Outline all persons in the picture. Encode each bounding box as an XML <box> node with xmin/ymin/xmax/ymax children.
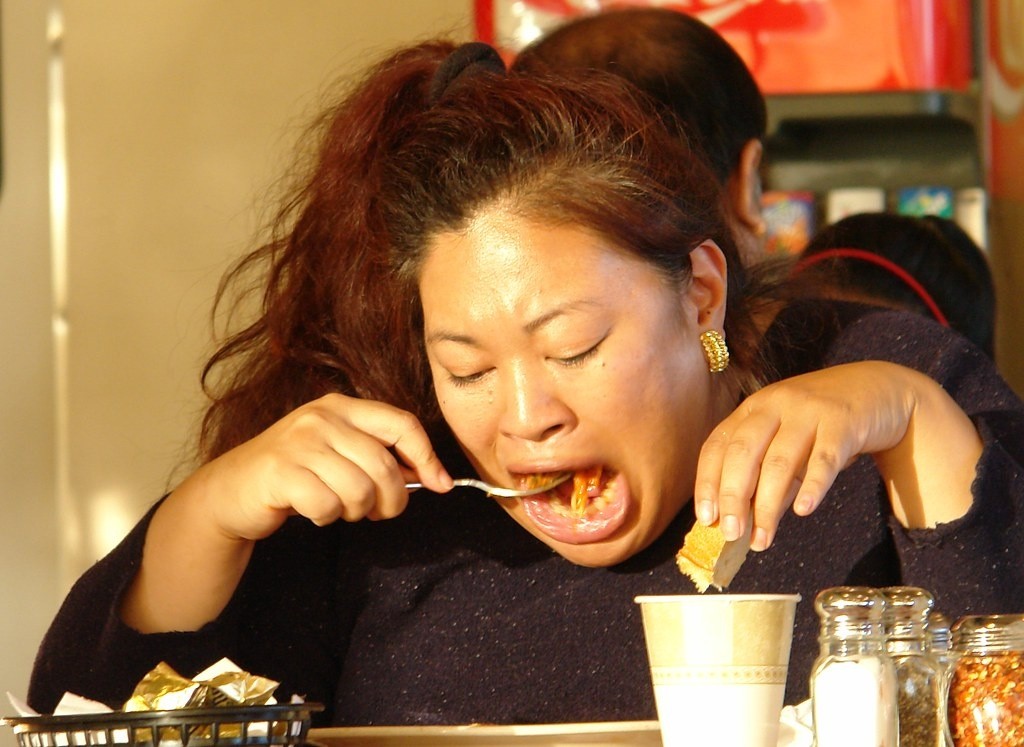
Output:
<box><xmin>26</xmin><ymin>8</ymin><xmax>1023</xmax><ymax>726</ymax></box>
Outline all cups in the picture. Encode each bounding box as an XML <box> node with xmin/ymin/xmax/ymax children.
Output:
<box><xmin>634</xmin><ymin>592</ymin><xmax>803</xmax><ymax>747</ymax></box>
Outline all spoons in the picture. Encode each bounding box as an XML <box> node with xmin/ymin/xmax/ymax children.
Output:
<box><xmin>401</xmin><ymin>472</ymin><xmax>576</xmax><ymax>500</ymax></box>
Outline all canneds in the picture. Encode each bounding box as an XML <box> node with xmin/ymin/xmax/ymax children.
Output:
<box><xmin>760</xmin><ymin>191</ymin><xmax>813</xmax><ymax>255</ymax></box>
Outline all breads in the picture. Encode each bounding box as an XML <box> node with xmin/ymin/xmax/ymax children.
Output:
<box><xmin>676</xmin><ymin>519</ymin><xmax>755</xmax><ymax>593</ymax></box>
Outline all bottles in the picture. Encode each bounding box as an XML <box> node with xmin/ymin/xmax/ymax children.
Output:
<box><xmin>880</xmin><ymin>586</ymin><xmax>939</xmax><ymax>747</ymax></box>
<box><xmin>811</xmin><ymin>588</ymin><xmax>899</xmax><ymax>747</ymax></box>
<box><xmin>945</xmin><ymin>615</ymin><xmax>1024</xmax><ymax>746</ymax></box>
